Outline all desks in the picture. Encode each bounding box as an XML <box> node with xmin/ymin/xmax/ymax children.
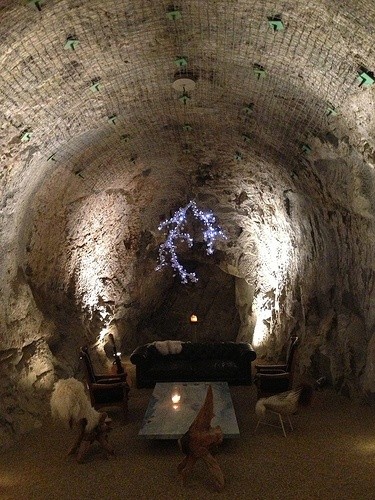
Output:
<box><xmin>137</xmin><ymin>382</ymin><xmax>240</xmax><ymax>440</ymax></box>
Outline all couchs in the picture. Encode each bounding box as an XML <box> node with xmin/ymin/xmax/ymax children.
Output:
<box><xmin>129</xmin><ymin>340</ymin><xmax>257</xmax><ymax>390</ymax></box>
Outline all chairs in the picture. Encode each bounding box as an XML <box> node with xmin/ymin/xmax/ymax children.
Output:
<box><xmin>77</xmin><ymin>346</ymin><xmax>131</xmax><ymax>425</ymax></box>
<box><xmin>254</xmin><ymin>387</ymin><xmax>303</xmax><ymax>439</ymax></box>
<box><xmin>254</xmin><ymin>336</ymin><xmax>302</xmax><ymax>401</ymax></box>
